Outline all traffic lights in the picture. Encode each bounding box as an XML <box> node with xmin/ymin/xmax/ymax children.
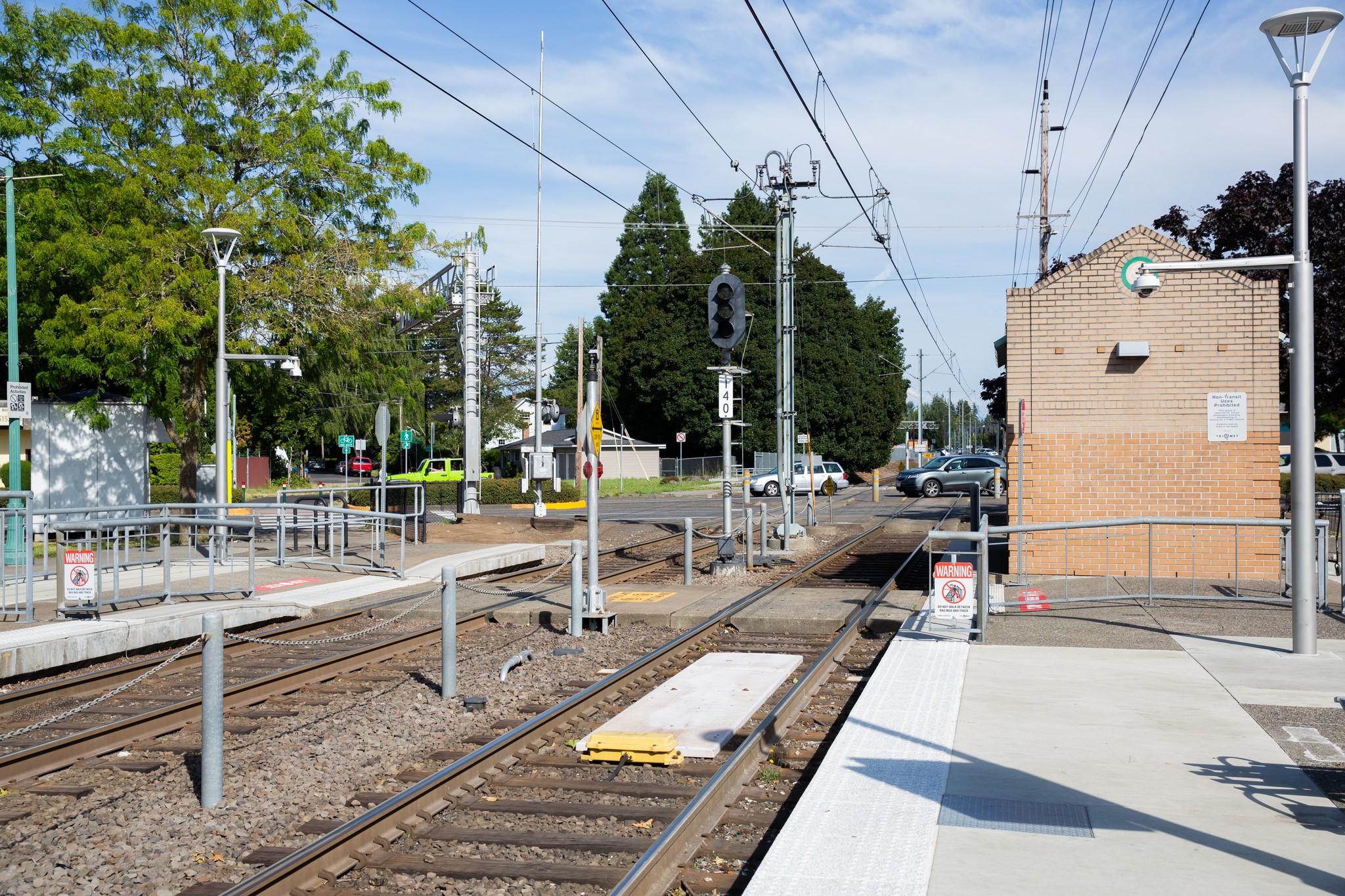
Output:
<box><xmin>708</xmin><ymin>273</ymin><xmax>745</xmax><ymax>349</ymax></box>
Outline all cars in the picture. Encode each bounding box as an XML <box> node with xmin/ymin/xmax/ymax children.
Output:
<box><xmin>305</xmin><ymin>457</ymin><xmax>325</xmax><ymax>470</ymax></box>
<box><xmin>335</xmin><ymin>456</ymin><xmax>380</xmax><ymax>477</ymax></box>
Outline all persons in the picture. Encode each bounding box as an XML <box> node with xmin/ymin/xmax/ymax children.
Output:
<box><xmin>428</xmin><ymin>461</ymin><xmax>445</xmax><ymax>471</ymax></box>
<box><xmin>794</xmin><ymin>465</ymin><xmax>804</xmax><ymax>474</ymax></box>
<box><xmin>954</xmin><ymin>462</ymin><xmax>962</xmax><ymax>469</ymax></box>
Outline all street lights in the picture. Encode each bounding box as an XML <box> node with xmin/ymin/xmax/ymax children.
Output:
<box><xmin>1259</xmin><ymin>5</ymin><xmax>1345</xmax><ymax>657</ymax></box>
<box><xmin>200</xmin><ymin>226</ymin><xmax>242</xmax><ymax>563</ymax></box>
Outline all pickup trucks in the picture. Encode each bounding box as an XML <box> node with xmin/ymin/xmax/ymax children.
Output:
<box><xmin>386</xmin><ymin>457</ymin><xmax>501</xmax><ymax>483</ymax></box>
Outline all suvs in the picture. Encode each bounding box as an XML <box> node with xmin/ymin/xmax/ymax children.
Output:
<box><xmin>742</xmin><ymin>460</ymin><xmax>849</xmax><ymax>497</ymax></box>
<box><xmin>895</xmin><ymin>454</ymin><xmax>1007</xmax><ymax>498</ymax></box>
<box><xmin>1278</xmin><ymin>450</ymin><xmax>1345</xmax><ymax>477</ymax></box>
